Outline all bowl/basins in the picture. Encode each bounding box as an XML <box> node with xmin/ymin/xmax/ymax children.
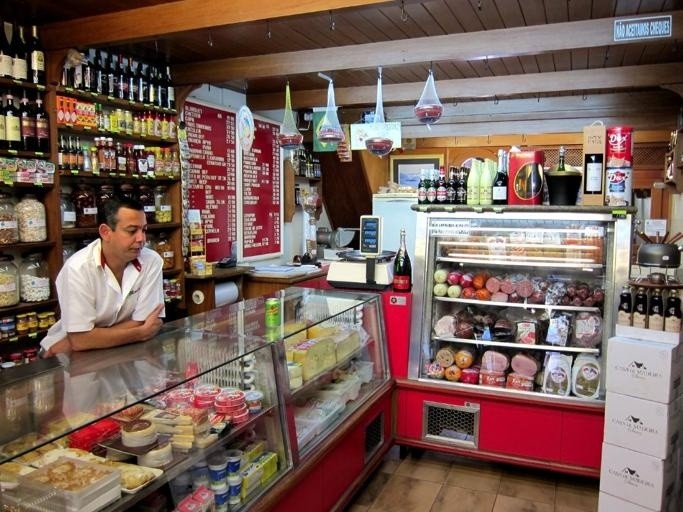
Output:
<box><xmin>414</xmin><ymin>105</ymin><xmax>442</xmax><ymax>123</ymax></box>
<box><xmin>276</xmin><ymin>134</ymin><xmax>301</xmax><ymax>149</ymax></box>
<box><xmin>365</xmin><ymin>139</ymin><xmax>392</xmax><ymax>155</ymax></box>
<box><xmin>319</xmin><ymin>129</ymin><xmax>343</xmax><ymax>146</ymax></box>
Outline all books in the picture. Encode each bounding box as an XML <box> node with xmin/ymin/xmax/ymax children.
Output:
<box><xmin>247</xmin><ymin>264</ymin><xmax>319</xmax><ymax>274</ymax></box>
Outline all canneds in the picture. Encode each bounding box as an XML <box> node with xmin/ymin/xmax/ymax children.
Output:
<box><xmin>103</xmin><ymin>108</ymin><xmax>141</xmax><ymax>134</ymax></box>
<box><xmin>0</xmin><ymin>311</ymin><xmax>56</xmax><ymax>336</ymax></box>
<box><xmin>0</xmin><ymin>251</ymin><xmax>49</xmax><ymax>307</ymax></box>
<box><xmin>61</xmin><ymin>182</ymin><xmax>173</xmax><ymax>230</ymax></box>
<box><xmin>0</xmin><ymin>190</ymin><xmax>48</xmax><ymax>244</ymax></box>
<box><xmin>63</xmin><ymin>232</ymin><xmax>176</xmax><ymax>268</ymax></box>
<box><xmin>265</xmin><ymin>298</ymin><xmax>279</xmax><ymax>329</ymax></box>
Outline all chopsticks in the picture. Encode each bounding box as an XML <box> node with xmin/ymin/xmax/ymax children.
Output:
<box><xmin>634</xmin><ymin>227</ymin><xmax>681</xmax><ymax>244</ymax></box>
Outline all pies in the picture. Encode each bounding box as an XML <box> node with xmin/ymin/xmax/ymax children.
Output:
<box><xmin>138</xmin><ymin>410</ymin><xmax>194</xmax><ymax>452</ymax></box>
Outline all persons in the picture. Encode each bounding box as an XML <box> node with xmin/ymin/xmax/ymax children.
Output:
<box><xmin>36</xmin><ymin>193</ymin><xmax>166</xmax><ymax>359</ymax></box>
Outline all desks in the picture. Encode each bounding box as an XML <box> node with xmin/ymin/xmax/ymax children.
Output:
<box><xmin>247</xmin><ymin>262</ymin><xmax>328</xmax><ymax>300</ymax></box>
<box><xmin>244</xmin><ymin>253</ymin><xmax>356</xmax><ymax>299</ymax></box>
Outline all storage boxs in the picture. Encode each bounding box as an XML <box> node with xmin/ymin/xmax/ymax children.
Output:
<box><xmin>600</xmin><ymin>443</ymin><xmax>682</xmax><ymax>511</ymax></box>
<box><xmin>602</xmin><ymin>391</ymin><xmax>682</xmax><ymax>459</ymax></box>
<box><xmin>598</xmin><ymin>491</ymin><xmax>654</xmax><ymax>512</ymax></box>
<box><xmin>605</xmin><ymin>336</ymin><xmax>682</xmax><ymax>404</ymax></box>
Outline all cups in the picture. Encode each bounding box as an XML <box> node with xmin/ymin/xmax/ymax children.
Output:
<box><xmin>545</xmin><ymin>164</ymin><xmax>581</xmax><ymax>205</ymax></box>
<box><xmin>172</xmin><ymin>446</ymin><xmax>244</xmax><ymax>512</ymax></box>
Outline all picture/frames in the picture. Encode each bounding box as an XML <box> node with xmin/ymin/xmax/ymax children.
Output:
<box><xmin>388</xmin><ymin>152</ymin><xmax>446</xmax><ymax>188</ymax></box>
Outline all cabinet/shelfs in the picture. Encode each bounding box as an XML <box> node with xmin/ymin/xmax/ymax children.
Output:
<box><xmin>186</xmin><ymin>263</ymin><xmax>256</xmax><ymax>316</ymax></box>
<box><xmin>161</xmin><ymin>284</ymin><xmax>394</xmax><ymax>467</ymax></box>
<box><xmin>615</xmin><ymin>230</ymin><xmax>683</xmax><ymax>346</ymax></box>
<box><xmin>52</xmin><ymin>43</ymin><xmax>186</xmax><ymax>320</ymax></box>
<box><xmin>0</xmin><ymin>332</ymin><xmax>294</xmax><ymax>512</ymax></box>
<box><xmin>0</xmin><ymin>25</ymin><xmax>55</xmax><ymax>368</ymax></box>
<box><xmin>284</xmin><ymin>159</ymin><xmax>324</xmax><ymax>224</ymax></box>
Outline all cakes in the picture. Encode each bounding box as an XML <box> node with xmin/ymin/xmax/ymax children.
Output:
<box><xmin>105</xmin><ymin>420</ymin><xmax>173</xmax><ymax>467</ymax></box>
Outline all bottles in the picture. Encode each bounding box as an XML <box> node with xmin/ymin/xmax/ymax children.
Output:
<box><xmin>391</xmin><ymin>227</ymin><xmax>414</xmax><ymax>291</ymax></box>
<box><xmin>555</xmin><ymin>144</ymin><xmax>567</xmax><ymax>171</ymax></box>
<box><xmin>0</xmin><ymin>191</ymin><xmax>50</xmax><ymax>307</ymax></box>
<box><xmin>0</xmin><ymin>21</ymin><xmax>47</xmax><ymax>86</ymax></box>
<box><xmin>0</xmin><ymin>88</ymin><xmax>51</xmax><ymax>149</ymax></box>
<box><xmin>92</xmin><ymin>101</ymin><xmax>178</xmax><ymax>140</ymax></box>
<box><xmin>616</xmin><ymin>284</ymin><xmax>681</xmax><ymax>334</ymax></box>
<box><xmin>413</xmin><ymin>146</ymin><xmax>506</xmax><ymax>204</ymax></box>
<box><xmin>59</xmin><ymin>46</ymin><xmax>176</xmax><ymax>108</ymax></box>
<box><xmin>292</xmin><ymin>184</ymin><xmax>300</xmax><ymax>205</ymax></box>
<box><xmin>293</xmin><ymin>150</ymin><xmax>321</xmax><ymax>177</ymax></box>
<box><xmin>514</xmin><ymin>162</ymin><xmax>540</xmax><ymax>198</ymax></box>
<box><xmin>60</xmin><ymin>183</ymin><xmax>175</xmax><ymax>271</ymax></box>
<box><xmin>56</xmin><ymin>131</ymin><xmax>180</xmax><ymax>179</ymax></box>
<box><xmin>57</xmin><ymin>97</ymin><xmax>76</xmax><ymax>122</ymax></box>
<box><xmin>584</xmin><ymin>154</ymin><xmax>601</xmax><ymax>193</ymax></box>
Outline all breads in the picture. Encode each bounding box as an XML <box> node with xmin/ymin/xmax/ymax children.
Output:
<box><xmin>1</xmin><ymin>411</ymin><xmax>154</xmax><ymax>510</ymax></box>
<box><xmin>264</xmin><ymin>321</ymin><xmax>361</xmax><ymax>390</ymax></box>
